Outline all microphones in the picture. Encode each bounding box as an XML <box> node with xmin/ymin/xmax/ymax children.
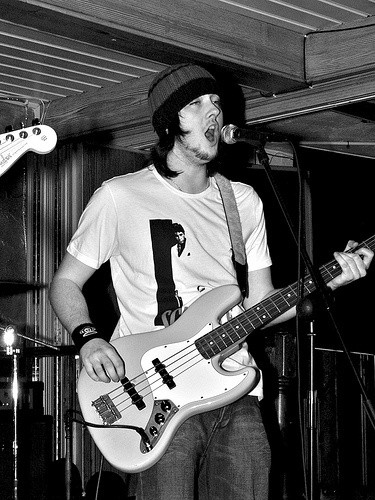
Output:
<box><xmin>220</xmin><ymin>124</ymin><xmax>290</xmax><ymax>145</ymax></box>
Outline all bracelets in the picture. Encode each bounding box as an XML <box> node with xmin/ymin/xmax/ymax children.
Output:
<box><xmin>71</xmin><ymin>322</ymin><xmax>102</xmax><ymax>351</ymax></box>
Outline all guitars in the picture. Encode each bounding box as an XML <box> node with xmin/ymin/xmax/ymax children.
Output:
<box><xmin>0</xmin><ymin>118</ymin><xmax>58</xmax><ymax>177</ymax></box>
<box><xmin>77</xmin><ymin>236</ymin><xmax>375</xmax><ymax>474</ymax></box>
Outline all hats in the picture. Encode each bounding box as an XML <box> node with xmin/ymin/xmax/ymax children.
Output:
<box><xmin>147</xmin><ymin>64</ymin><xmax>218</xmax><ymax>129</ymax></box>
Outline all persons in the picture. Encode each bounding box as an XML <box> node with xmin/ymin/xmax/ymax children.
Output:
<box><xmin>48</xmin><ymin>64</ymin><xmax>374</xmax><ymax>500</ymax></box>
